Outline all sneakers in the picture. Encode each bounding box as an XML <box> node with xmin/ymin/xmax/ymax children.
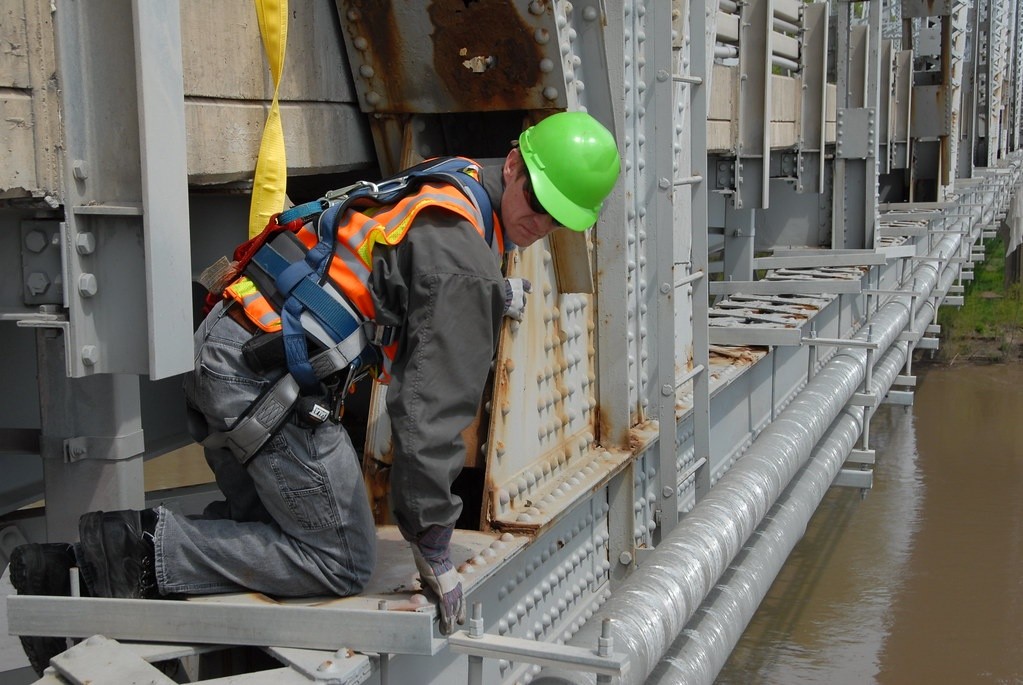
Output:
<box><xmin>78</xmin><ymin>510</ymin><xmax>155</xmax><ymax>599</ymax></box>
<box><xmin>7</xmin><ymin>542</ymin><xmax>75</xmax><ymax>678</ymax></box>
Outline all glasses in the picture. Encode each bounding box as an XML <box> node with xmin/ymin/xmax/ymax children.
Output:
<box><xmin>522</xmin><ymin>164</ymin><xmax>567</xmax><ymax>228</ymax></box>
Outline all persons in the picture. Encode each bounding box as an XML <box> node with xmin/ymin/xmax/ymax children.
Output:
<box><xmin>7</xmin><ymin>109</ymin><xmax>622</xmax><ymax>679</ymax></box>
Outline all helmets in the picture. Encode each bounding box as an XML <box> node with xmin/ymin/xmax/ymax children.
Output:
<box><xmin>519</xmin><ymin>112</ymin><xmax>621</xmax><ymax>233</ymax></box>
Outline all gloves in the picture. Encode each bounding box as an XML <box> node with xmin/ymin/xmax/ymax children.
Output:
<box><xmin>393</xmin><ymin>511</ymin><xmax>467</xmax><ymax>636</ymax></box>
<box><xmin>500</xmin><ymin>277</ymin><xmax>533</xmax><ymax>324</ymax></box>
